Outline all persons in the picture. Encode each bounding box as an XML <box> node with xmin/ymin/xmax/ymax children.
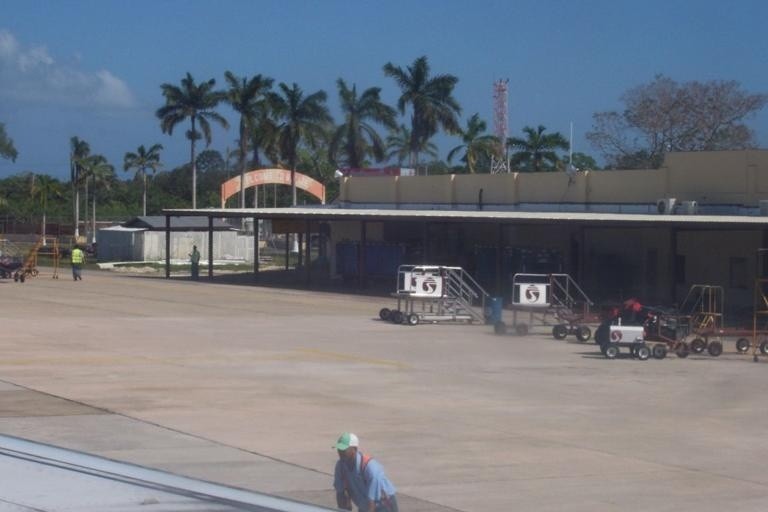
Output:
<box><xmin>69</xmin><ymin>242</ymin><xmax>84</xmax><ymax>281</ymax></box>
<box><xmin>188</xmin><ymin>245</ymin><xmax>201</xmax><ymax>278</ymax></box>
<box><xmin>333</xmin><ymin>431</ymin><xmax>398</xmax><ymax>511</ymax></box>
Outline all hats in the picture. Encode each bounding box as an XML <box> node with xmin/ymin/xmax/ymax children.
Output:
<box><xmin>332</xmin><ymin>432</ymin><xmax>359</xmax><ymax>450</ymax></box>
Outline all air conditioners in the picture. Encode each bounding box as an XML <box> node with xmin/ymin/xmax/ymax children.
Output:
<box><xmin>654</xmin><ymin>198</ymin><xmax>698</xmax><ymax>215</ymax></box>
